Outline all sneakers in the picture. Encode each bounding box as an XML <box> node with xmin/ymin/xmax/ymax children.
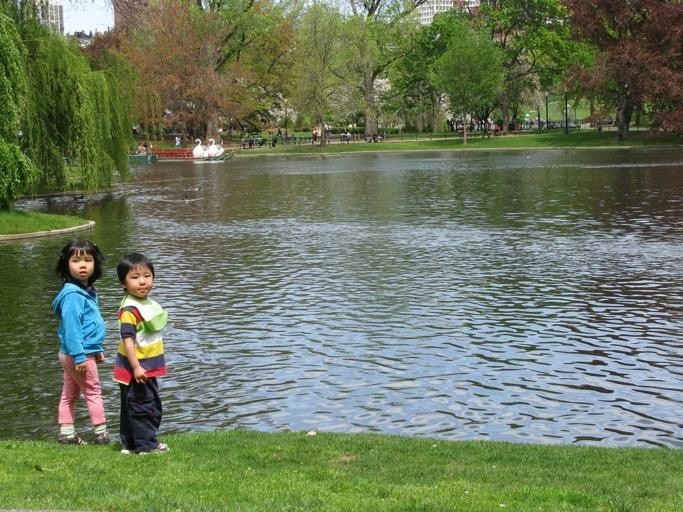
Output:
<box><xmin>58</xmin><ymin>432</ymin><xmax>87</xmax><ymax>446</ymax></box>
<box><xmin>121</xmin><ymin>442</ymin><xmax>170</xmax><ymax>455</ymax></box>
<box><xmin>94</xmin><ymin>434</ymin><xmax>111</xmax><ymax>445</ymax></box>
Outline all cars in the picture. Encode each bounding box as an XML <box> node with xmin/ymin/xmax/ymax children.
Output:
<box><xmin>454</xmin><ymin>120</ymin><xmax>472</xmax><ymax>131</ymax></box>
<box><xmin>597</xmin><ymin>117</ymin><xmax>613</xmax><ymax>125</ymax></box>
<box><xmin>548</xmin><ymin>122</ymin><xmax>577</xmax><ymax>129</ymax></box>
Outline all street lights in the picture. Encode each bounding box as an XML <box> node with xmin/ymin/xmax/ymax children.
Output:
<box><xmin>520</xmin><ymin>92</ymin><xmax>524</xmax><ymax>130</ymax></box>
<box><xmin>624</xmin><ymin>84</ymin><xmax>630</xmax><ymax>137</ymax></box>
<box><xmin>283</xmin><ymin>96</ymin><xmax>288</xmax><ymax>144</ymax></box>
<box><xmin>564</xmin><ymin>87</ymin><xmax>569</xmax><ymax>135</ymax></box>
<box><xmin>545</xmin><ymin>92</ymin><xmax>549</xmax><ymax>127</ymax></box>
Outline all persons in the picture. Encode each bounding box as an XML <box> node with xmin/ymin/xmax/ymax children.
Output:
<box><xmin>50</xmin><ymin>237</ymin><xmax>116</xmax><ymax>445</ymax></box>
<box><xmin>111</xmin><ymin>254</ymin><xmax>172</xmax><ymax>456</ymax></box>
<box><xmin>128</xmin><ymin>117</ymin><xmax>620</xmax><ymax>155</ymax></box>
